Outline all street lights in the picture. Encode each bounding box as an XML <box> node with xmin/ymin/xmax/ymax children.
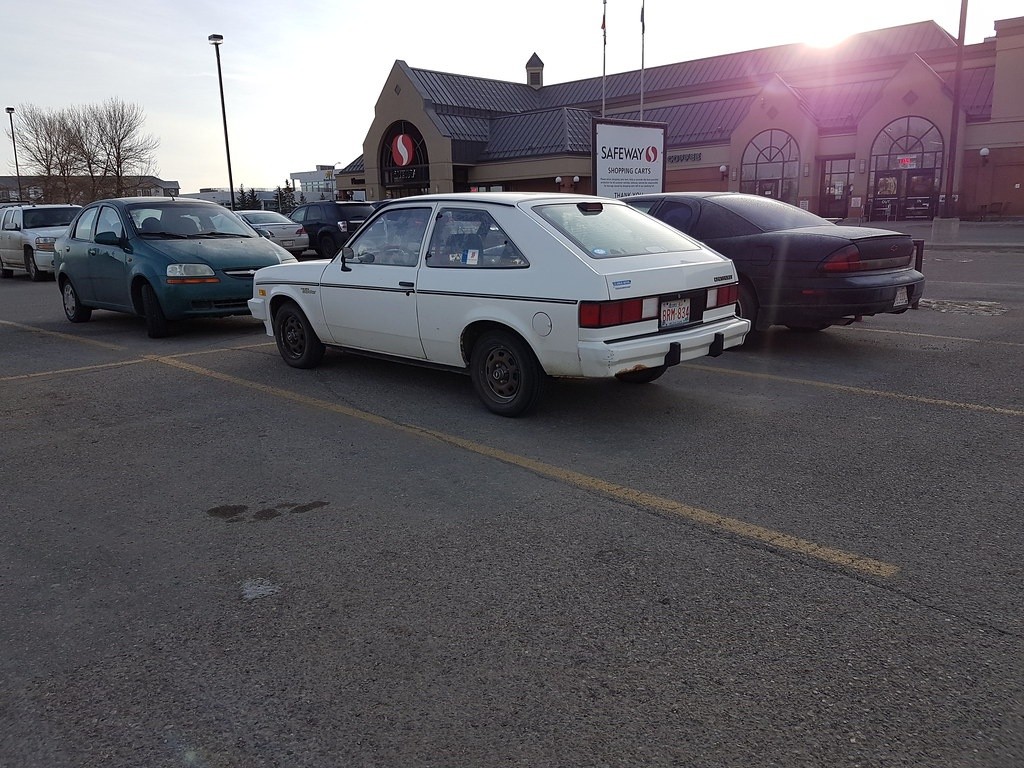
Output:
<box><xmin>6</xmin><ymin>107</ymin><xmax>23</xmax><ymax>202</ymax></box>
<box><xmin>208</xmin><ymin>33</ymin><xmax>235</xmax><ymax>212</ymax></box>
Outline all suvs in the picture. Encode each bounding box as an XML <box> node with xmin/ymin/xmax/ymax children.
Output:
<box><xmin>284</xmin><ymin>199</ymin><xmax>387</xmax><ymax>260</ymax></box>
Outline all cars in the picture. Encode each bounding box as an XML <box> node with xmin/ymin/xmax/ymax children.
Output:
<box><xmin>244</xmin><ymin>193</ymin><xmax>751</xmax><ymax>420</ymax></box>
<box><xmin>480</xmin><ymin>191</ymin><xmax>924</xmax><ymax>349</ymax></box>
<box><xmin>52</xmin><ymin>197</ymin><xmax>299</xmax><ymax>339</ymax></box>
<box><xmin>198</xmin><ymin>210</ymin><xmax>309</xmax><ymax>258</ymax></box>
<box><xmin>371</xmin><ymin>200</ymin><xmax>481</xmax><ymax>243</ymax></box>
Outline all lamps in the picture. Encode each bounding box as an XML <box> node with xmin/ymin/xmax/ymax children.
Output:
<box><xmin>859</xmin><ymin>159</ymin><xmax>865</xmax><ymax>173</ymax></box>
<box><xmin>570</xmin><ymin>176</ymin><xmax>579</xmax><ymax>190</ymax></box>
<box><xmin>369</xmin><ymin>187</ymin><xmax>373</xmax><ymax>197</ymax></box>
<box><xmin>804</xmin><ymin>163</ymin><xmax>809</xmax><ymax>177</ymax></box>
<box><xmin>435</xmin><ymin>186</ymin><xmax>439</xmax><ymax>194</ymax></box>
<box><xmin>556</xmin><ymin>176</ymin><xmax>563</xmax><ymax>192</ymax></box>
<box><xmin>346</xmin><ymin>191</ymin><xmax>353</xmax><ymax>200</ymax></box>
<box><xmin>979</xmin><ymin>148</ymin><xmax>990</xmax><ymax>166</ymax></box>
<box><xmin>720</xmin><ymin>165</ymin><xmax>728</xmax><ymax>179</ymax></box>
<box><xmin>334</xmin><ymin>190</ymin><xmax>341</xmax><ymax>200</ymax></box>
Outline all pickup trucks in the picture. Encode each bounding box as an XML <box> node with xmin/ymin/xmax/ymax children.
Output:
<box><xmin>0</xmin><ymin>205</ymin><xmax>85</xmax><ymax>281</ymax></box>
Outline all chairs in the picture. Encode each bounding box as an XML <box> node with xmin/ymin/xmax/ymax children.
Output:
<box><xmin>446</xmin><ymin>235</ymin><xmax>487</xmax><ymax>268</ymax></box>
<box><xmin>174</xmin><ymin>216</ymin><xmax>199</xmax><ymax>233</ymax></box>
<box><xmin>141</xmin><ymin>216</ymin><xmax>161</xmax><ymax>232</ymax></box>
